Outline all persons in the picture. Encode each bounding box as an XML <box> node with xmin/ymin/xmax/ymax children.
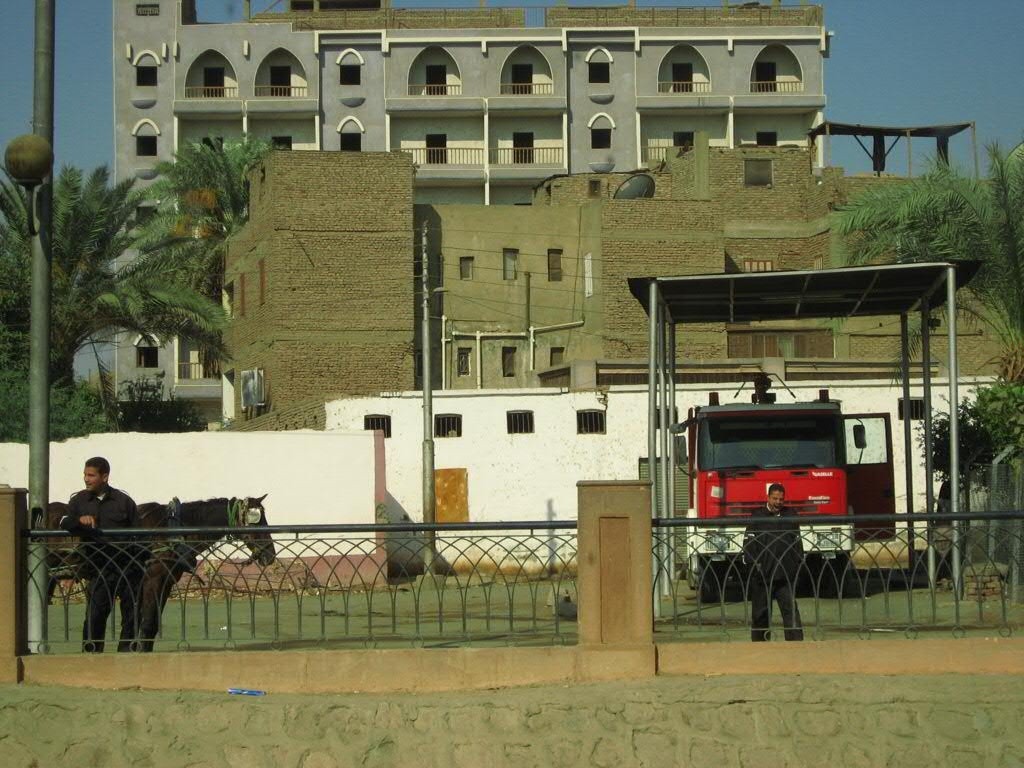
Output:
<box><xmin>742</xmin><ymin>484</ymin><xmax>805</xmax><ymax>642</ymax></box>
<box><xmin>57</xmin><ymin>456</ymin><xmax>146</xmax><ymax>652</ymax></box>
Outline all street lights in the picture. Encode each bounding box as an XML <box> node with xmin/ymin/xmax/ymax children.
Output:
<box><xmin>4</xmin><ymin>135</ymin><xmax>57</xmax><ymax>655</ymax></box>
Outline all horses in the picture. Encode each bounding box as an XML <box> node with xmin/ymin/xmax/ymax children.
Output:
<box><xmin>27</xmin><ymin>493</ymin><xmax>276</xmax><ymax>652</ymax></box>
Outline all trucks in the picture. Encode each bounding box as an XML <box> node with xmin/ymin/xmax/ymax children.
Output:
<box><xmin>668</xmin><ymin>372</ymin><xmax>899</xmax><ymax>603</ymax></box>
